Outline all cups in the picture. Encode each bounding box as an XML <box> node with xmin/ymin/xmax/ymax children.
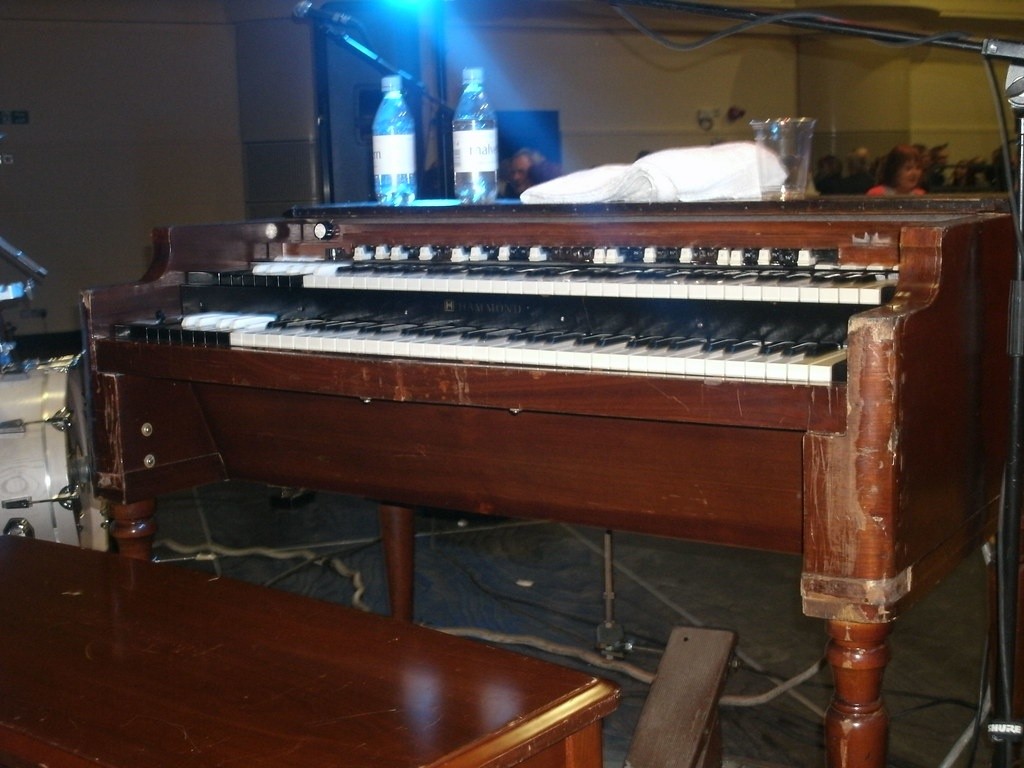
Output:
<box><xmin>749</xmin><ymin>117</ymin><xmax>817</xmax><ymax>200</ymax></box>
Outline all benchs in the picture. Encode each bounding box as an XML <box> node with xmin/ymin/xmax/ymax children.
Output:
<box><xmin>0</xmin><ymin>533</ymin><xmax>624</xmax><ymax>768</ymax></box>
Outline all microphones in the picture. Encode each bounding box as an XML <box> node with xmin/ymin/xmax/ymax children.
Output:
<box><xmin>291</xmin><ymin>0</ymin><xmax>358</xmax><ymax>28</ymax></box>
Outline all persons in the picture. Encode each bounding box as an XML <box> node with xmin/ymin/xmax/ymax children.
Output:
<box><xmin>500</xmin><ymin>146</ymin><xmax>564</xmax><ymax>199</ymax></box>
<box><xmin>812</xmin><ymin>140</ymin><xmax>1021</xmax><ymax>197</ymax></box>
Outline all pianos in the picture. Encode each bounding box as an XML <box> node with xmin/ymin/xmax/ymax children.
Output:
<box><xmin>78</xmin><ymin>191</ymin><xmax>1022</xmax><ymax>768</ymax></box>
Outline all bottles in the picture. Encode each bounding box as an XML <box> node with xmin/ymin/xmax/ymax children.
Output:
<box><xmin>453</xmin><ymin>67</ymin><xmax>499</xmax><ymax>203</ymax></box>
<box><xmin>371</xmin><ymin>76</ymin><xmax>418</xmax><ymax>204</ymax></box>
